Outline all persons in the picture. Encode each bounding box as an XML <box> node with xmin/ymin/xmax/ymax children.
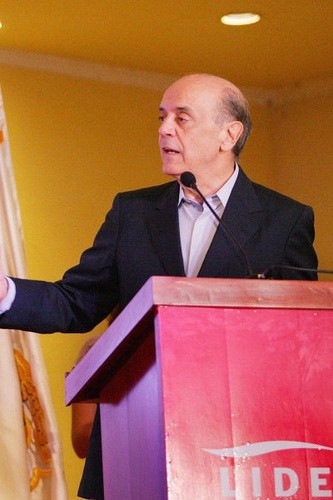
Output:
<box><xmin>0</xmin><ymin>72</ymin><xmax>321</xmax><ymax>500</ymax></box>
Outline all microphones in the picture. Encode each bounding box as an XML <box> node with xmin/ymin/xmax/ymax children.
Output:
<box><xmin>263</xmin><ymin>264</ymin><xmax>333</xmax><ymax>276</ymax></box>
<box><xmin>180</xmin><ymin>170</ymin><xmax>257</xmax><ymax>279</ymax></box>
